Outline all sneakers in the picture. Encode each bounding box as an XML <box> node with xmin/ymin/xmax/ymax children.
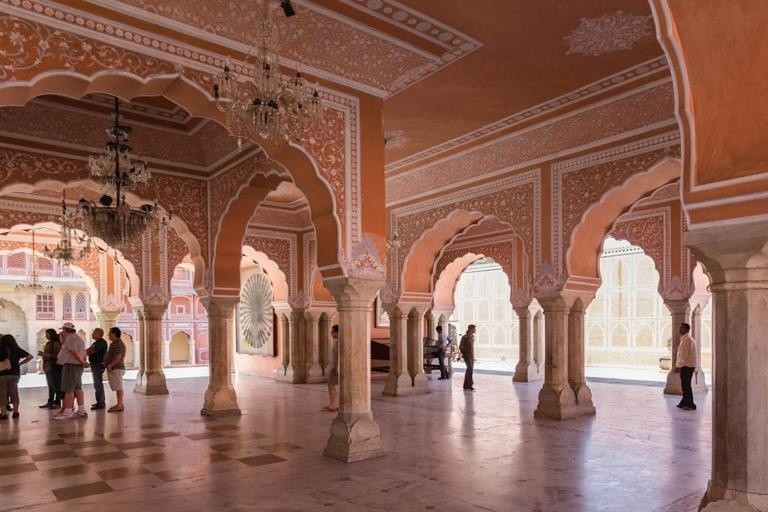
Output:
<box><xmin>320</xmin><ymin>406</ymin><xmax>338</xmax><ymax>412</ymax></box>
<box><xmin>0</xmin><ymin>404</ymin><xmax>19</xmax><ymax>420</ymax></box>
<box><xmin>39</xmin><ymin>402</ymin><xmax>124</xmax><ymax>420</ymax></box>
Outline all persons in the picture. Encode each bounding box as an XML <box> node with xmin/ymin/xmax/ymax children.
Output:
<box><xmin>37</xmin><ymin>328</ymin><xmax>61</xmax><ymax>409</ymax></box>
<box><xmin>675</xmin><ymin>322</ymin><xmax>699</xmax><ymax>410</ymax></box>
<box><xmin>102</xmin><ymin>326</ymin><xmax>127</xmax><ymax>411</ymax></box>
<box><xmin>322</xmin><ymin>324</ymin><xmax>338</xmax><ymax>411</ymax></box>
<box><xmin>53</xmin><ymin>322</ymin><xmax>90</xmax><ymax>420</ymax></box>
<box><xmin>459</xmin><ymin>323</ymin><xmax>477</xmax><ymax>391</ymax></box>
<box><xmin>0</xmin><ymin>334</ymin><xmax>33</xmax><ymax>419</ymax></box>
<box><xmin>85</xmin><ymin>327</ymin><xmax>107</xmax><ymax>409</ymax></box>
<box><xmin>6</xmin><ymin>396</ymin><xmax>13</xmax><ymax>412</ymax></box>
<box><xmin>436</xmin><ymin>325</ymin><xmax>452</xmax><ymax>380</ymax></box>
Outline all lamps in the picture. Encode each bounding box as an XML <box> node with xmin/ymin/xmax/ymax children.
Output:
<box><xmin>197</xmin><ymin>1</ymin><xmax>321</xmax><ymax>154</ymax></box>
<box><xmin>11</xmin><ymin>96</ymin><xmax>182</xmax><ymax>296</ymax></box>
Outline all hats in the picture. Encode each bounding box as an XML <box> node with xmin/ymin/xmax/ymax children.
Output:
<box><xmin>59</xmin><ymin>323</ymin><xmax>76</xmax><ymax>331</ymax></box>
